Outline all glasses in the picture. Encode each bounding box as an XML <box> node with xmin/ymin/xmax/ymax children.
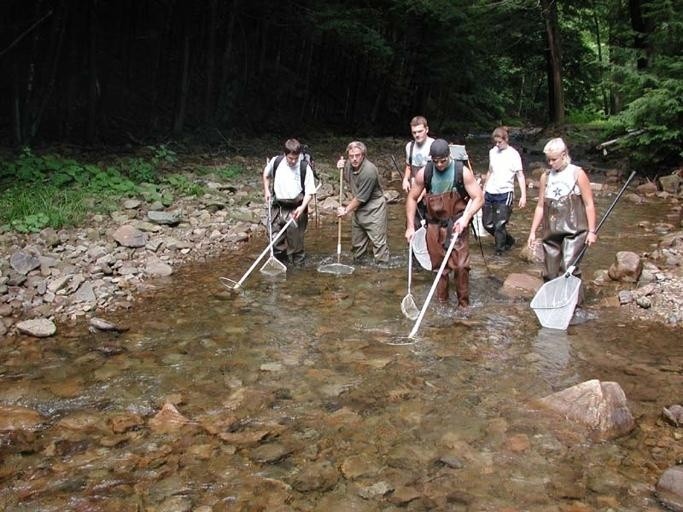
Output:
<box><xmin>432</xmin><ymin>159</ymin><xmax>446</xmax><ymax>163</ymax></box>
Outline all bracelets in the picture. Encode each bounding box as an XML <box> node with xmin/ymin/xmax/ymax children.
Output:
<box><xmin>588</xmin><ymin>230</ymin><xmax>595</xmax><ymax>234</ymax></box>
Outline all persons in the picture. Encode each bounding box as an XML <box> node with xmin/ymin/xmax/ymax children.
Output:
<box><xmin>261</xmin><ymin>137</ymin><xmax>317</xmax><ymax>268</ymax></box>
<box><xmin>335</xmin><ymin>140</ymin><xmax>390</xmax><ymax>266</ymax></box>
<box><xmin>399</xmin><ymin>114</ymin><xmax>436</xmax><ymax>234</ymax></box>
<box><xmin>480</xmin><ymin>124</ymin><xmax>527</xmax><ymax>257</ymax></box>
<box><xmin>403</xmin><ymin>138</ymin><xmax>485</xmax><ymax>310</ymax></box>
<box><xmin>526</xmin><ymin>137</ymin><xmax>598</xmax><ymax>327</ymax></box>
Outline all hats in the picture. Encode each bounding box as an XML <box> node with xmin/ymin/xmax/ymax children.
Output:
<box><xmin>429</xmin><ymin>140</ymin><xmax>449</xmax><ymax>158</ymax></box>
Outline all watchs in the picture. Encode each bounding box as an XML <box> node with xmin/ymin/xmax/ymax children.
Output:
<box><xmin>343</xmin><ymin>207</ymin><xmax>348</xmax><ymax>216</ymax></box>
